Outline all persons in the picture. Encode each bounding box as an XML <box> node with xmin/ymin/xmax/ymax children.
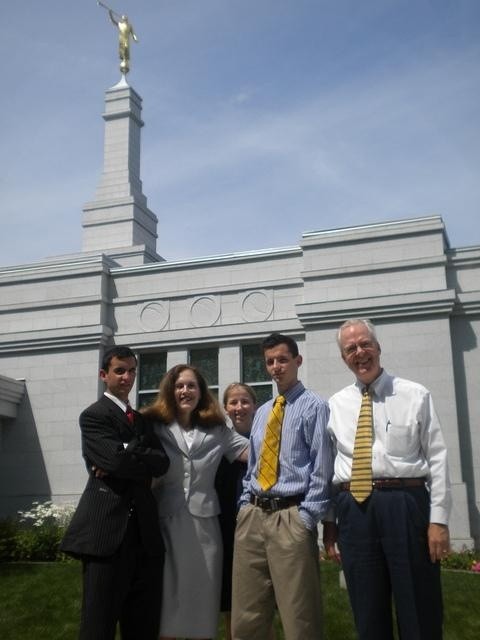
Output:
<box><xmin>107</xmin><ymin>8</ymin><xmax>141</xmax><ymax>71</ymax></box>
<box><xmin>133</xmin><ymin>361</ymin><xmax>250</xmax><ymax>640</ymax></box>
<box><xmin>212</xmin><ymin>381</ymin><xmax>258</xmax><ymax>639</ymax></box>
<box><xmin>318</xmin><ymin>316</ymin><xmax>454</xmax><ymax>640</ymax></box>
<box><xmin>229</xmin><ymin>332</ymin><xmax>334</xmax><ymax>640</ymax></box>
<box><xmin>57</xmin><ymin>345</ymin><xmax>174</xmax><ymax>640</ymax></box>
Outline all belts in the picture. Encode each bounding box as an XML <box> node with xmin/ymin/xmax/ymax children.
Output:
<box><xmin>340</xmin><ymin>478</ymin><xmax>425</xmax><ymax>491</ymax></box>
<box><xmin>249</xmin><ymin>493</ymin><xmax>305</xmax><ymax>514</ymax></box>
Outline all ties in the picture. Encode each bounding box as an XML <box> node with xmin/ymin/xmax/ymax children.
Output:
<box><xmin>259</xmin><ymin>395</ymin><xmax>286</xmax><ymax>491</ymax></box>
<box><xmin>350</xmin><ymin>387</ymin><xmax>373</xmax><ymax>504</ymax></box>
<box><xmin>126</xmin><ymin>405</ymin><xmax>134</xmax><ymax>424</ymax></box>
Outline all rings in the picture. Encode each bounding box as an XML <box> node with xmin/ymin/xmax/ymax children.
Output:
<box><xmin>443</xmin><ymin>549</ymin><xmax>448</xmax><ymax>553</ymax></box>
